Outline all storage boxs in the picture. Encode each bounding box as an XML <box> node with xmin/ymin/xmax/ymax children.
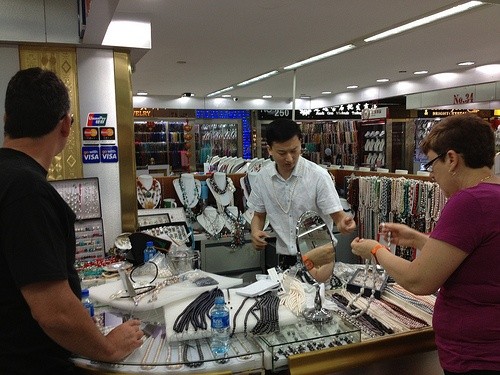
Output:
<box><xmin>138</xmin><ymin>213</ymin><xmax>172</xmax><ymax>225</ymax></box>
<box><xmin>347</xmin><ymin>268</ymin><xmax>390</xmax><ymax>300</ymax></box>
<box><xmin>48</xmin><ymin>177</ymin><xmax>106</xmax><ymax>262</ymax></box>
<box><xmin>139</xmin><ymin>220</ymin><xmax>192</xmax><ymax>247</ymax></box>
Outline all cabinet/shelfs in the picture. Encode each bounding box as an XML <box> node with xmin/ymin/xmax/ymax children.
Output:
<box><xmin>134</xmin><ymin>122</ymin><xmax>190</xmax><ymax>172</ymax></box>
<box><xmin>201</xmin><ymin>227</ymin><xmax>358</xmax><ymax>273</ymax></box>
<box><xmin>353</xmin><ymin>120</ymin><xmax>386</xmax><ymax>168</ymax></box>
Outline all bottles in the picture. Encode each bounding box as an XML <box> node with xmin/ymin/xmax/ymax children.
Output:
<box><xmin>212</xmin><ymin>297</ymin><xmax>229</xmax><ymax>353</ymax></box>
<box><xmin>79</xmin><ymin>289</ymin><xmax>96</xmax><ymax>323</ymax></box>
<box><xmin>143</xmin><ymin>242</ymin><xmax>157</xmax><ymax>265</ymax></box>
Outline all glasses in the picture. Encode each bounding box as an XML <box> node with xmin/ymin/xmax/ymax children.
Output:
<box><xmin>424</xmin><ymin>150</ymin><xmax>460</xmax><ymax>172</ymax></box>
<box><xmin>60</xmin><ymin>112</ymin><xmax>75</xmax><ymax>126</ymax></box>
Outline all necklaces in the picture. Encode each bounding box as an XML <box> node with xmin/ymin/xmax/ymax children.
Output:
<box><xmin>480</xmin><ymin>174</ymin><xmax>495</xmax><ymax>182</ymax></box>
<box><xmin>47</xmin><ymin>121</ymin><xmax>452</xmax><ymax>371</ymax></box>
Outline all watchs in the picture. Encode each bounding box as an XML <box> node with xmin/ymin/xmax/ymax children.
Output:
<box><xmin>371</xmin><ymin>245</ymin><xmax>392</xmax><ymax>265</ymax></box>
<box><xmin>302</xmin><ymin>255</ymin><xmax>314</xmax><ymax>271</ymax></box>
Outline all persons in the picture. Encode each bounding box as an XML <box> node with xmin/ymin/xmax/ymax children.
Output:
<box><xmin>0</xmin><ymin>66</ymin><xmax>145</xmax><ymax>375</ymax></box>
<box><xmin>251</xmin><ymin>119</ymin><xmax>356</xmax><ymax>272</ymax></box>
<box><xmin>351</xmin><ymin>113</ymin><xmax>500</xmax><ymax>375</ymax></box>
